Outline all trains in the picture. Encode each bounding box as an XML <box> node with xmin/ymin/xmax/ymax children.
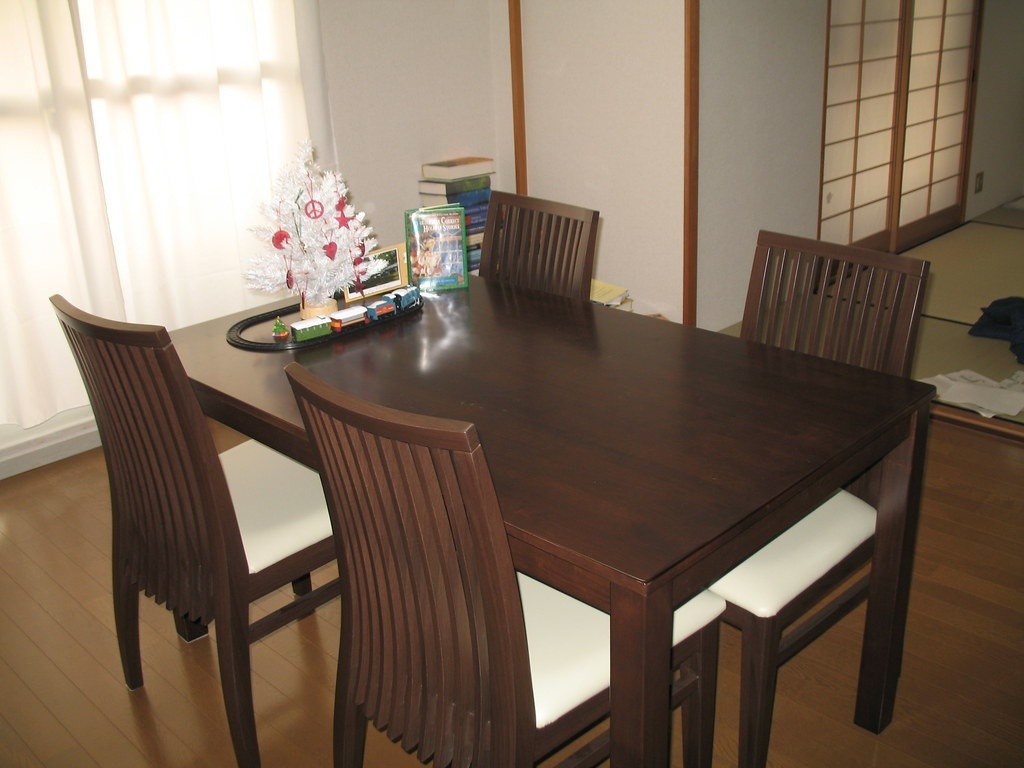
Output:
<box><xmin>291</xmin><ymin>286</ymin><xmax>421</xmax><ymax>342</ymax></box>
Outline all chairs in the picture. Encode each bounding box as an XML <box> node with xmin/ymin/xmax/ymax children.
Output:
<box><xmin>702</xmin><ymin>228</ymin><xmax>931</xmax><ymax>768</ymax></box>
<box><xmin>283</xmin><ymin>361</ymin><xmax>726</xmax><ymax>768</ymax></box>
<box><xmin>478</xmin><ymin>190</ymin><xmax>600</xmax><ymax>301</ymax></box>
<box><xmin>50</xmin><ymin>293</ymin><xmax>342</xmax><ymax>768</ymax></box>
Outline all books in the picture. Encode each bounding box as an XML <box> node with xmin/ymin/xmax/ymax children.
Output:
<box><xmin>406</xmin><ymin>202</ymin><xmax>469</xmax><ymax>289</ymax></box>
<box><xmin>419</xmin><ymin>157</ymin><xmax>505</xmax><ymax>277</ymax></box>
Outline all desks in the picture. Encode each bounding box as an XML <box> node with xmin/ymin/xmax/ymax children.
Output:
<box><xmin>168</xmin><ymin>273</ymin><xmax>937</xmax><ymax>768</ymax></box>
